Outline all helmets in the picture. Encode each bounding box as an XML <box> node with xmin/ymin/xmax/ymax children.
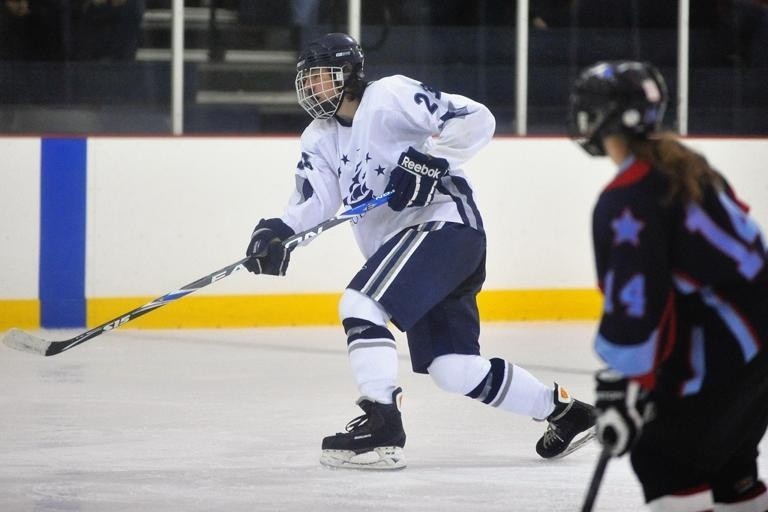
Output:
<box><xmin>568</xmin><ymin>61</ymin><xmax>666</xmax><ymax>156</ymax></box>
<box><xmin>294</xmin><ymin>33</ymin><xmax>365</xmax><ymax>121</ymax></box>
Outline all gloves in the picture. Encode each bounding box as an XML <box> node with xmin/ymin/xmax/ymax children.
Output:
<box><xmin>594</xmin><ymin>370</ymin><xmax>642</xmax><ymax>454</ymax></box>
<box><xmin>386</xmin><ymin>148</ymin><xmax>448</xmax><ymax>210</ymax></box>
<box><xmin>246</xmin><ymin>217</ymin><xmax>295</xmax><ymax>276</ymax></box>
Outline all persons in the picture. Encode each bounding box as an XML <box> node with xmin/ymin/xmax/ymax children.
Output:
<box><xmin>244</xmin><ymin>32</ymin><xmax>603</xmax><ymax>461</ymax></box>
<box><xmin>567</xmin><ymin>55</ymin><xmax>768</xmax><ymax>512</ymax></box>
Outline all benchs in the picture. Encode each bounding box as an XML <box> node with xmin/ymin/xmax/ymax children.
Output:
<box><xmin>139</xmin><ymin>0</ymin><xmax>768</xmax><ymax>129</ymax></box>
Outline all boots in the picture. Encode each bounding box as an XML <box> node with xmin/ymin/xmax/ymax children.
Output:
<box><xmin>536</xmin><ymin>385</ymin><xmax>599</xmax><ymax>456</ymax></box>
<box><xmin>321</xmin><ymin>388</ymin><xmax>405</xmax><ymax>448</ymax></box>
